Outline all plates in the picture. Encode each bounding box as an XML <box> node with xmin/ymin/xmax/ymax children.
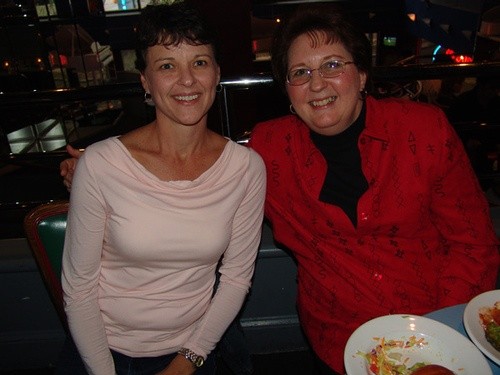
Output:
<box><xmin>344</xmin><ymin>314</ymin><xmax>493</xmax><ymax>375</ymax></box>
<box><xmin>463</xmin><ymin>290</ymin><xmax>500</xmax><ymax>366</ymax></box>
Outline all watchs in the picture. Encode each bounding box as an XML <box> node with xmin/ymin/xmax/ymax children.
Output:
<box><xmin>176</xmin><ymin>350</ymin><xmax>206</xmax><ymax>368</ymax></box>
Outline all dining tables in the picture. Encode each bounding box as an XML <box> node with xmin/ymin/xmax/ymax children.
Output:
<box><xmin>422</xmin><ymin>303</ymin><xmax>500</xmax><ymax>374</ymax></box>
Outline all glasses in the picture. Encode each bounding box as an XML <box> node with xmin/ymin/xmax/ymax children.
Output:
<box><xmin>286</xmin><ymin>60</ymin><xmax>357</xmax><ymax>86</ymax></box>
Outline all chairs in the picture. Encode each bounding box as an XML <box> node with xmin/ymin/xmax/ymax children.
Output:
<box><xmin>23</xmin><ymin>201</ymin><xmax>69</xmax><ymax>335</ymax></box>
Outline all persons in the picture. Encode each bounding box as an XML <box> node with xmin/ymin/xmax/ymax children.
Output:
<box><xmin>62</xmin><ymin>5</ymin><xmax>267</xmax><ymax>375</ymax></box>
<box><xmin>61</xmin><ymin>10</ymin><xmax>500</xmax><ymax>375</ymax></box>
